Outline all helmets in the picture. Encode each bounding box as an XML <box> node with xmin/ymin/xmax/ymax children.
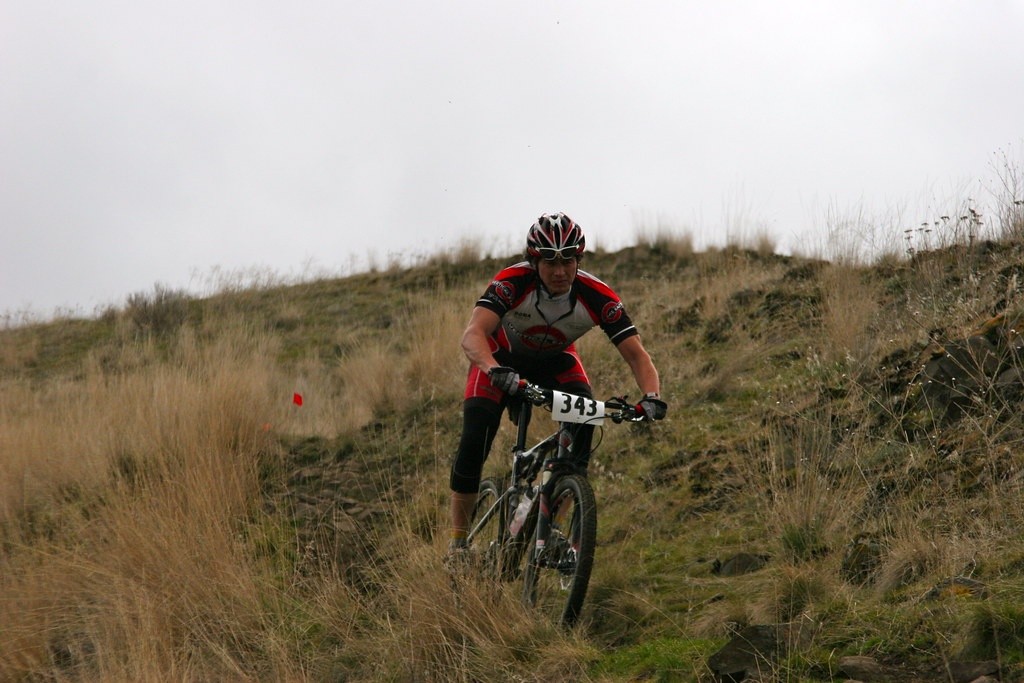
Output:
<box><xmin>526</xmin><ymin>211</ymin><xmax>585</xmax><ymax>256</ymax></box>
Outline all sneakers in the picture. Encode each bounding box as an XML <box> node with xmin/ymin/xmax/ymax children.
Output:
<box><xmin>443</xmin><ymin>546</ymin><xmax>472</xmax><ymax>580</ymax></box>
<box><xmin>526</xmin><ymin>533</ymin><xmax>578</xmax><ymax>574</ymax></box>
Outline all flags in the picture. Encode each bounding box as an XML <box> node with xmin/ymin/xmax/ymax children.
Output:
<box><xmin>292</xmin><ymin>392</ymin><xmax>302</xmax><ymax>406</ymax></box>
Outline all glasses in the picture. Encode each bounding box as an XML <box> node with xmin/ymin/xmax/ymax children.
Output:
<box><xmin>531</xmin><ymin>243</ymin><xmax>582</xmax><ymax>261</ymax></box>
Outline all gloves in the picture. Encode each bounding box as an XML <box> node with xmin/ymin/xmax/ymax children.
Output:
<box><xmin>637</xmin><ymin>392</ymin><xmax>666</xmax><ymax>422</ymax></box>
<box><xmin>490</xmin><ymin>366</ymin><xmax>520</xmax><ymax>396</ymax></box>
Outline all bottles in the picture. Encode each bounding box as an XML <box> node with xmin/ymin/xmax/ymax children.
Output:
<box><xmin>511</xmin><ymin>484</ymin><xmax>541</xmax><ymax>538</ymax></box>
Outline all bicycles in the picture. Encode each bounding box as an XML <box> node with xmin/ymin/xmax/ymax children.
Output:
<box><xmin>459</xmin><ymin>375</ymin><xmax>659</xmax><ymax>634</ymax></box>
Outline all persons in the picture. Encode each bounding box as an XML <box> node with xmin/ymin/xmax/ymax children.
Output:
<box><xmin>440</xmin><ymin>213</ymin><xmax>662</xmax><ymax>579</ymax></box>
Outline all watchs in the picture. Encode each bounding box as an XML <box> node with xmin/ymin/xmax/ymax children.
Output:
<box><xmin>643</xmin><ymin>392</ymin><xmax>658</xmax><ymax>400</ymax></box>
